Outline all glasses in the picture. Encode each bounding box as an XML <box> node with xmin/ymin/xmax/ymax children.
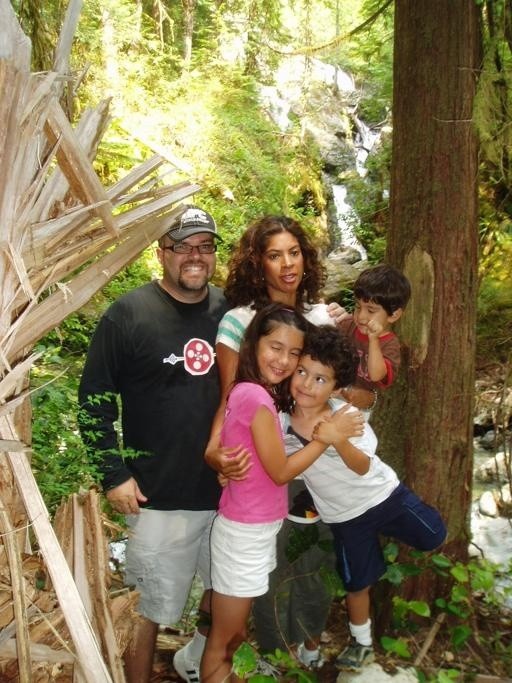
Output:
<box><xmin>164</xmin><ymin>243</ymin><xmax>217</xmax><ymax>254</ymax></box>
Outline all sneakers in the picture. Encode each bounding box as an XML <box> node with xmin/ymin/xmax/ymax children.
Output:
<box><xmin>298</xmin><ymin>643</ymin><xmax>325</xmax><ymax>671</ymax></box>
<box><xmin>334</xmin><ymin>636</ymin><xmax>376</xmax><ymax>673</ymax></box>
<box><xmin>286</xmin><ymin>489</ymin><xmax>321</xmax><ymax>525</ymax></box>
<box><xmin>172</xmin><ymin>638</ymin><xmax>203</xmax><ymax>683</ymax></box>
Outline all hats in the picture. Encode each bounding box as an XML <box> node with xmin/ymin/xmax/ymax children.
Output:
<box><xmin>166</xmin><ymin>204</ymin><xmax>224</xmax><ymax>243</ymax></box>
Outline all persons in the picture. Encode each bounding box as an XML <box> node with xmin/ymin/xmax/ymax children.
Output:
<box><xmin>215</xmin><ymin>323</ymin><xmax>447</xmax><ymax>671</ymax></box>
<box><xmin>199</xmin><ymin>303</ymin><xmax>367</xmax><ymax>683</ymax></box>
<box><xmin>314</xmin><ymin>265</ymin><xmax>416</xmax><ymax>388</ymax></box>
<box><xmin>201</xmin><ymin>212</ymin><xmax>381</xmax><ymax>672</ymax></box>
<box><xmin>77</xmin><ymin>207</ymin><xmax>350</xmax><ymax>682</ymax></box>
